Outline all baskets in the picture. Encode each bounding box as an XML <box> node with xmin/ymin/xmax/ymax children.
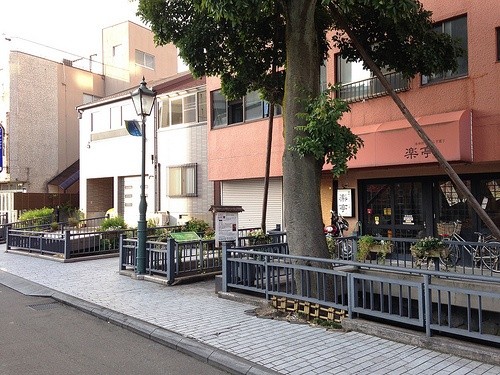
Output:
<box><xmin>437</xmin><ymin>223</ymin><xmax>462</xmax><ymax>236</ymax></box>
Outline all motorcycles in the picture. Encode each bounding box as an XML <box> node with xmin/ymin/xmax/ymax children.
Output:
<box><xmin>323</xmin><ymin>209</ymin><xmax>357</xmax><ymax>260</ymax></box>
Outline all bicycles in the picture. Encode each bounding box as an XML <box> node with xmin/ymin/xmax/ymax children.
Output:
<box><xmin>438</xmin><ymin>219</ymin><xmax>499</xmax><ymax>275</ymax></box>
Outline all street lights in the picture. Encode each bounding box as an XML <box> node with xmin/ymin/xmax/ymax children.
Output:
<box><xmin>130</xmin><ymin>75</ymin><xmax>157</xmax><ymax>275</ymax></box>
